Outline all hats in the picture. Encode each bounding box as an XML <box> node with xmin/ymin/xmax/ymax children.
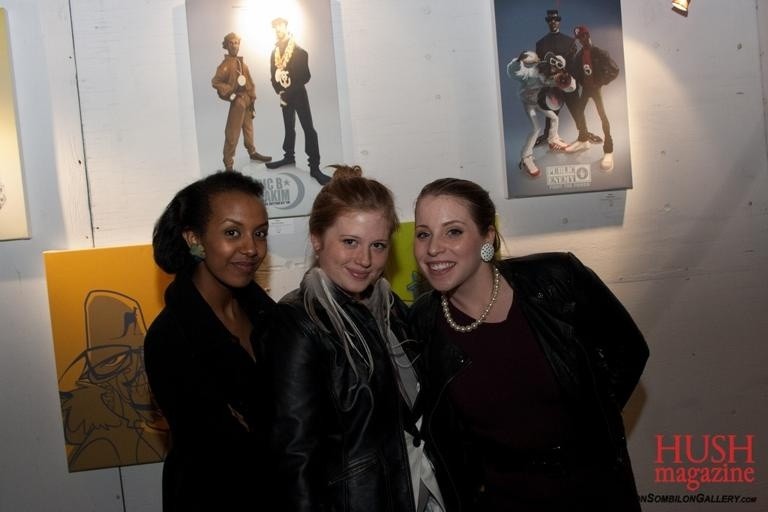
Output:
<box><xmin>223</xmin><ymin>32</ymin><xmax>240</xmax><ymax>49</ymax></box>
<box><xmin>574</xmin><ymin>26</ymin><xmax>589</xmax><ymax>39</ymax></box>
<box><xmin>271</xmin><ymin>18</ymin><xmax>288</xmax><ymax>26</ymax></box>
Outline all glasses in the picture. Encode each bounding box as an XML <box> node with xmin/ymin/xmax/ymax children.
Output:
<box><xmin>550</xmin><ymin>58</ymin><xmax>563</xmax><ymax>70</ymax></box>
<box><xmin>545</xmin><ymin>15</ymin><xmax>562</xmax><ymax>23</ymax></box>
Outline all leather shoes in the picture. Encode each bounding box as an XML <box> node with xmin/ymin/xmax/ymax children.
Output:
<box><xmin>250</xmin><ymin>153</ymin><xmax>272</xmax><ymax>161</ymax></box>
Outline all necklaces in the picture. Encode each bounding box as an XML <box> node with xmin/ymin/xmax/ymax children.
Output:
<box><xmin>273</xmin><ymin>36</ymin><xmax>296</xmax><ymax>89</ymax></box>
<box><xmin>440</xmin><ymin>265</ymin><xmax>500</xmax><ymax>332</ymax></box>
<box><xmin>229</xmin><ymin>53</ymin><xmax>247</xmax><ymax>86</ymax></box>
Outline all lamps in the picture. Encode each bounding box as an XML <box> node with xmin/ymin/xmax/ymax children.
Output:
<box><xmin>672</xmin><ymin>1</ymin><xmax>688</xmax><ymax>13</ymax></box>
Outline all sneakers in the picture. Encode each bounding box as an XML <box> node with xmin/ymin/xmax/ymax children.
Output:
<box><xmin>548</xmin><ymin>139</ymin><xmax>568</xmax><ymax>152</ymax></box>
<box><xmin>310</xmin><ymin>167</ymin><xmax>331</xmax><ymax>186</ymax></box>
<box><xmin>524</xmin><ymin>156</ymin><xmax>540</xmax><ymax>177</ymax></box>
<box><xmin>535</xmin><ymin>135</ymin><xmax>549</xmax><ymax>146</ymax></box>
<box><xmin>578</xmin><ymin>132</ymin><xmax>602</xmax><ymax>143</ymax></box>
<box><xmin>601</xmin><ymin>153</ymin><xmax>613</xmax><ymax>170</ymax></box>
<box><xmin>265</xmin><ymin>157</ymin><xmax>295</xmax><ymax>169</ymax></box>
<box><xmin>564</xmin><ymin>140</ymin><xmax>590</xmax><ymax>153</ymax></box>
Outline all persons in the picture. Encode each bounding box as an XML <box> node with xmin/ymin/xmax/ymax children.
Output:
<box><xmin>252</xmin><ymin>162</ymin><xmax>445</xmax><ymax>510</ymax></box>
<box><xmin>563</xmin><ymin>25</ymin><xmax>621</xmax><ymax>171</ymax></box>
<box><xmin>263</xmin><ymin>19</ymin><xmax>333</xmax><ymax>186</ymax></box>
<box><xmin>533</xmin><ymin>8</ymin><xmax>602</xmax><ymax>142</ymax></box>
<box><xmin>505</xmin><ymin>51</ymin><xmax>576</xmax><ymax>176</ymax></box>
<box><xmin>144</xmin><ymin>169</ymin><xmax>277</xmax><ymax>510</ymax></box>
<box><xmin>211</xmin><ymin>32</ymin><xmax>273</xmax><ymax>176</ymax></box>
<box><xmin>406</xmin><ymin>176</ymin><xmax>650</xmax><ymax>509</ymax></box>
